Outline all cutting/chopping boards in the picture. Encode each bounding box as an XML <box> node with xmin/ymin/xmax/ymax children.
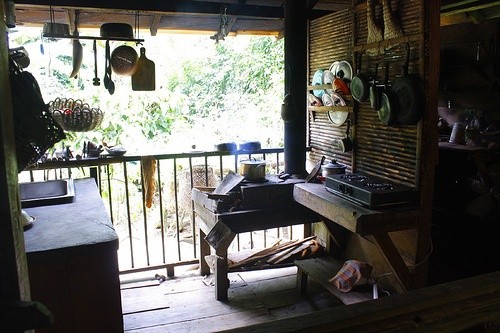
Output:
<box><xmin>131</xmin><ymin>47</ymin><xmax>156</xmax><ymax>91</ymax></box>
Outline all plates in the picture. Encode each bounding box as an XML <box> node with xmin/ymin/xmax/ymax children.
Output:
<box><xmin>86</xmin><ymin>150</ymin><xmax>104</xmax><ymax>156</ymax></box>
<box><xmin>110</xmin><ymin>151</ymin><xmax>126</xmax><ymax>155</ymax></box>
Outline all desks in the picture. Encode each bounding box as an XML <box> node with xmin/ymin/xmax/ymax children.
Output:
<box><xmin>21</xmin><ymin>177</ymin><xmax>124</xmax><ymax>333</ymax></box>
<box><xmin>293</xmin><ymin>182</ymin><xmax>421</xmax><ymax>294</ymax></box>
<box><xmin>193</xmin><ymin>202</ymin><xmax>322</xmax><ymax>301</ymax></box>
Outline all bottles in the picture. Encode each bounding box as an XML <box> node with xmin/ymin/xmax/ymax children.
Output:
<box><xmin>447</xmin><ymin>122</ymin><xmax>466</xmax><ymax>144</ymax></box>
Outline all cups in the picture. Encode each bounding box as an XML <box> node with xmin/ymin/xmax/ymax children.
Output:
<box><xmin>464</xmin><ymin>124</ymin><xmax>481</xmax><ymax>146</ymax></box>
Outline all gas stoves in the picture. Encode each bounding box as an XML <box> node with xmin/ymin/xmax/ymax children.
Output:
<box><xmin>325</xmin><ymin>173</ymin><xmax>415</xmax><ymax>208</ymax></box>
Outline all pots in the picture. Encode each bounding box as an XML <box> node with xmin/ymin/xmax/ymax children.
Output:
<box><xmin>240</xmin><ymin>158</ymin><xmax>266</xmax><ymax>179</ymax></box>
<box><xmin>321</xmin><ymin>159</ymin><xmax>347</xmax><ymax>177</ymax></box>
<box><xmin>349</xmin><ymin>43</ymin><xmax>420</xmax><ymax>125</ymax></box>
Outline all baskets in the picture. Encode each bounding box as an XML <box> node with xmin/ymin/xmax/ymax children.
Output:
<box><xmin>47</xmin><ymin>97</ymin><xmax>104</xmax><ymax>131</ymax></box>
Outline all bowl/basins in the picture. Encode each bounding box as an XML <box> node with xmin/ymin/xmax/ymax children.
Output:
<box><xmin>99</xmin><ymin>23</ymin><xmax>133</xmax><ymax>39</ymax></box>
<box><xmin>43</xmin><ymin>22</ymin><xmax>70</xmax><ymax>36</ymax></box>
